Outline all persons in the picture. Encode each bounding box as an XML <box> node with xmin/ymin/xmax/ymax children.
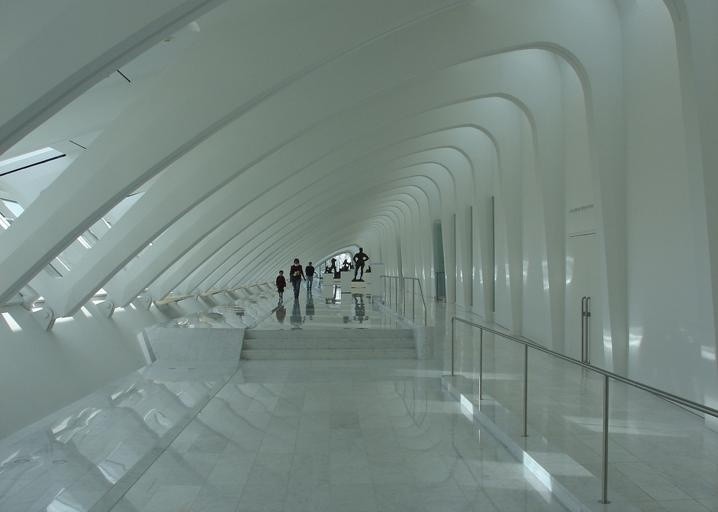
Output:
<box><xmin>276</xmin><ymin>271</ymin><xmax>286</xmax><ymax>295</ymax></box>
<box><xmin>289</xmin><ymin>258</ymin><xmax>305</xmax><ymax>298</ymax></box>
<box><xmin>324</xmin><ymin>247</ymin><xmax>371</xmax><ymax>280</ymax></box>
<box><xmin>306</xmin><ymin>292</ymin><xmax>315</xmax><ymax>320</ymax></box>
<box><xmin>271</xmin><ymin>295</ymin><xmax>287</xmax><ymax>323</ymax></box>
<box><xmin>305</xmin><ymin>261</ymin><xmax>314</xmax><ymax>292</ymax></box>
<box><xmin>288</xmin><ymin>299</ymin><xmax>306</xmax><ymax>326</ymax></box>
<box><xmin>352</xmin><ymin>297</ymin><xmax>369</xmax><ymax>323</ymax></box>
